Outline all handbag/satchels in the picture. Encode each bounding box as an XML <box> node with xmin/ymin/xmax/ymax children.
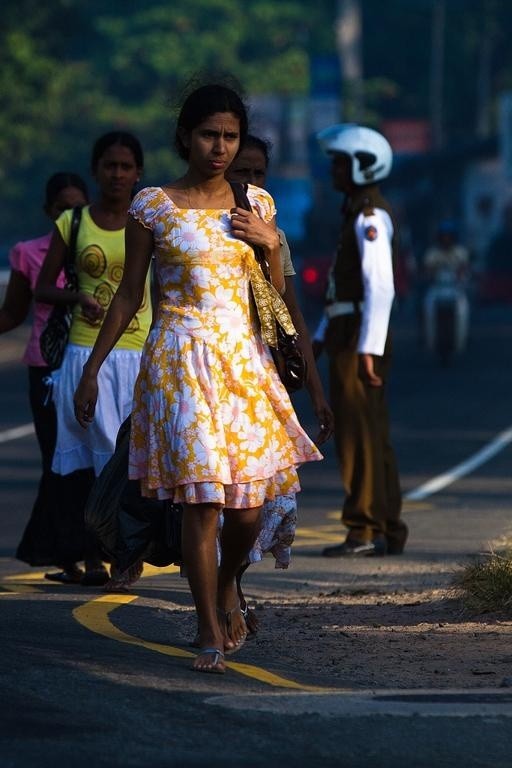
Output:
<box><xmin>39</xmin><ymin>207</ymin><xmax>83</xmax><ymax>367</ymax></box>
<box><xmin>273</xmin><ymin>321</ymin><xmax>308</xmax><ymax>394</ymax></box>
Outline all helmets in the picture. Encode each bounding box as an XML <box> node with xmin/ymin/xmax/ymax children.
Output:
<box><xmin>324</xmin><ymin>125</ymin><xmax>393</xmax><ymax>186</ymax></box>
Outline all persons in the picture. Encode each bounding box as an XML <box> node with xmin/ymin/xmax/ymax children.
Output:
<box><xmin>310</xmin><ymin>122</ymin><xmax>408</xmax><ymax>557</ymax></box>
<box><xmin>417</xmin><ymin>219</ymin><xmax>474</xmax><ymax>354</ymax></box>
<box><xmin>34</xmin><ymin>128</ymin><xmax>153</xmax><ymax>591</ymax></box>
<box><xmin>0</xmin><ymin>170</ymin><xmax>110</xmax><ymax>589</ymax></box>
<box><xmin>216</xmin><ymin>129</ymin><xmax>335</xmax><ymax>637</ymax></box>
<box><xmin>72</xmin><ymin>64</ymin><xmax>324</xmax><ymax>674</ymax></box>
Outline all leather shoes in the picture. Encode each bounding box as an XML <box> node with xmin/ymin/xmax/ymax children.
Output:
<box><xmin>322</xmin><ymin>539</ymin><xmax>403</xmax><ymax>558</ymax></box>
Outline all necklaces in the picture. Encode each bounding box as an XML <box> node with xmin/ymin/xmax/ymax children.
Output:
<box><xmin>184</xmin><ymin>182</ymin><xmax>230</xmax><ymax>209</ymax></box>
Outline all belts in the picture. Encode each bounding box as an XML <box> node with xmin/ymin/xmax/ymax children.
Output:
<box><xmin>324</xmin><ymin>301</ymin><xmax>366</xmax><ymax>319</ymax></box>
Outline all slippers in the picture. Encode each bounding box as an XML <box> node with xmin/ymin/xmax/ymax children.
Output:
<box><xmin>191</xmin><ymin>600</ymin><xmax>247</xmax><ymax>674</ymax></box>
<box><xmin>44</xmin><ymin>560</ymin><xmax>142</xmax><ymax>590</ymax></box>
